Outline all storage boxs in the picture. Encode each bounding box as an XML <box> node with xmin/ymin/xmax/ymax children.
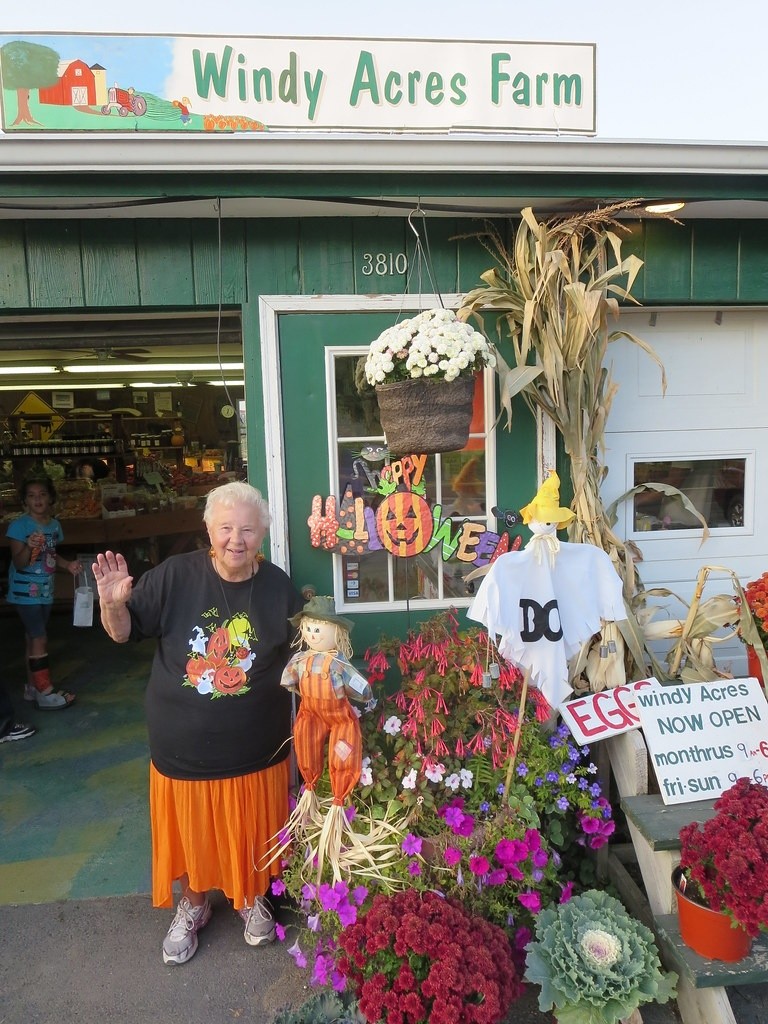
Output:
<box><xmin>202</xmin><ymin>448</ymin><xmax>228</xmax><ymax>472</ymax></box>
<box><xmin>98</xmin><ymin>481</ymin><xmax>136</xmax><ymax>520</ymax></box>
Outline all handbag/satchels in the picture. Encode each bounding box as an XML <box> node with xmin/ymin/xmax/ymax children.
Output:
<box><xmin>72</xmin><ymin>570</ymin><xmax>93</xmax><ymax>626</ymax></box>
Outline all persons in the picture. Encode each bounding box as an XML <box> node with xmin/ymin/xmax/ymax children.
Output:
<box><xmin>280</xmin><ymin>597</ymin><xmax>374</xmax><ymax>808</ymax></box>
<box><xmin>634</xmin><ymin>460</ymin><xmax>714</xmax><ymax>529</ymax></box>
<box><xmin>466</xmin><ymin>470</ymin><xmax>626</xmax><ymax>711</ymax></box>
<box><xmin>0</xmin><ymin>683</ymin><xmax>36</xmax><ymax>744</ymax></box>
<box><xmin>6</xmin><ymin>479</ymin><xmax>83</xmax><ymax>712</ymax></box>
<box><xmin>90</xmin><ymin>480</ymin><xmax>310</xmax><ymax>967</ymax></box>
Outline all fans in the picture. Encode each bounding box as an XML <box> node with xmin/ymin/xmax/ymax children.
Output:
<box><xmin>152</xmin><ymin>372</ymin><xmax>215</xmax><ymax>387</ymax></box>
<box><xmin>56</xmin><ymin>347</ymin><xmax>153</xmax><ymax>363</ymax></box>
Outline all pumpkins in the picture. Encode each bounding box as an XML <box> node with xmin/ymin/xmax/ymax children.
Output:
<box><xmin>171</xmin><ymin>435</ymin><xmax>184</xmax><ymax>446</ymax></box>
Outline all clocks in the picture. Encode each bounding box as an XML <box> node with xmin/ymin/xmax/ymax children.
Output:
<box><xmin>221</xmin><ymin>405</ymin><xmax>234</xmax><ymax>419</ymax></box>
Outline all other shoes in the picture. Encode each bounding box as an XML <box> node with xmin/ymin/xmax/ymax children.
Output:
<box><xmin>35</xmin><ymin>686</ymin><xmax>76</xmax><ymax>710</ymax></box>
<box><xmin>24</xmin><ymin>683</ymin><xmax>36</xmax><ymax>700</ymax></box>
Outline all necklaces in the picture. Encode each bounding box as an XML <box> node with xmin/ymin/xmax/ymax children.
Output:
<box><xmin>213</xmin><ymin>556</ymin><xmax>255</xmax><ymax>659</ymax></box>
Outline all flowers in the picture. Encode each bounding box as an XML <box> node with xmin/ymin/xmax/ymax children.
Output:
<box><xmin>677</xmin><ymin>778</ymin><xmax>768</xmax><ymax>937</ymax></box>
<box><xmin>318</xmin><ymin>889</ymin><xmax>518</xmax><ymax>1024</ymax></box>
<box><xmin>363</xmin><ymin>306</ymin><xmax>498</xmax><ymax>384</ymax></box>
<box><xmin>269</xmin><ymin>599</ymin><xmax>616</xmax><ymax>990</ymax></box>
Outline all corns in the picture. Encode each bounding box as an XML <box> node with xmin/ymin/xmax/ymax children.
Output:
<box><xmin>643</xmin><ymin>620</ymin><xmax>688</xmax><ymax>640</ymax></box>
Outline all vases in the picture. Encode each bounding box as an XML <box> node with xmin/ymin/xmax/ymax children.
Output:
<box><xmin>375</xmin><ymin>375</ymin><xmax>476</xmax><ymax>454</ymax></box>
<box><xmin>670</xmin><ymin>864</ymin><xmax>755</xmax><ymax>962</ymax></box>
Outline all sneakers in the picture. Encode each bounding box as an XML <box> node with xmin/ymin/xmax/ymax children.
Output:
<box><xmin>0</xmin><ymin>718</ymin><xmax>36</xmax><ymax>743</ymax></box>
<box><xmin>163</xmin><ymin>895</ymin><xmax>212</xmax><ymax>965</ymax></box>
<box><xmin>238</xmin><ymin>895</ymin><xmax>277</xmax><ymax>946</ymax></box>
